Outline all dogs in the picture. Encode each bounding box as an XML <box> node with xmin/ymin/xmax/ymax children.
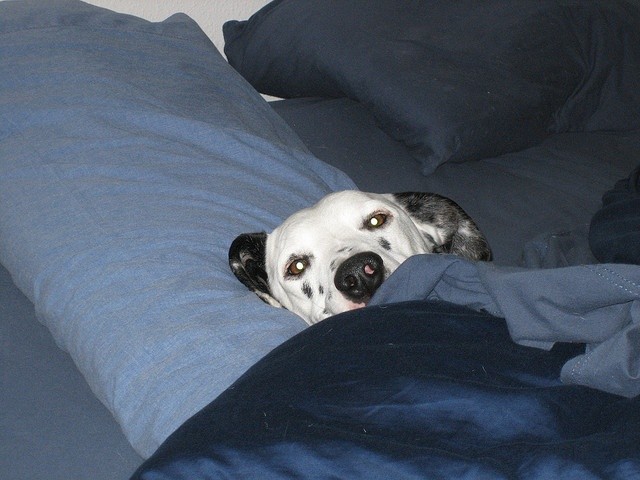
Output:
<box><xmin>228</xmin><ymin>190</ymin><xmax>493</xmax><ymax>331</ymax></box>
